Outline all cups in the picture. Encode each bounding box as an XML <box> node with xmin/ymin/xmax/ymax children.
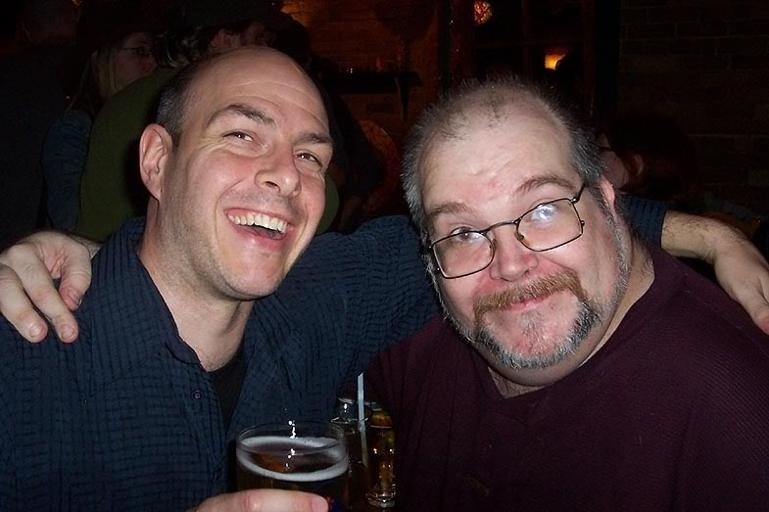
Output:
<box><xmin>229</xmin><ymin>419</ymin><xmax>351</xmax><ymax>512</ymax></box>
<box><xmin>331</xmin><ymin>411</ymin><xmax>398</xmax><ymax>512</ymax></box>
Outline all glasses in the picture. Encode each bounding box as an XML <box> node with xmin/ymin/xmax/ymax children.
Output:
<box><xmin>425</xmin><ymin>178</ymin><xmax>589</xmax><ymax>279</ymax></box>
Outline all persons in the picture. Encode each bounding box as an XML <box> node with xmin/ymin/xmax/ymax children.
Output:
<box><xmin>74</xmin><ymin>3</ymin><xmax>346</xmax><ymax>249</ymax></box>
<box><xmin>36</xmin><ymin>26</ymin><xmax>162</xmax><ymax>233</ymax></box>
<box><xmin>0</xmin><ymin>2</ymin><xmax>83</xmax><ymax>242</ymax></box>
